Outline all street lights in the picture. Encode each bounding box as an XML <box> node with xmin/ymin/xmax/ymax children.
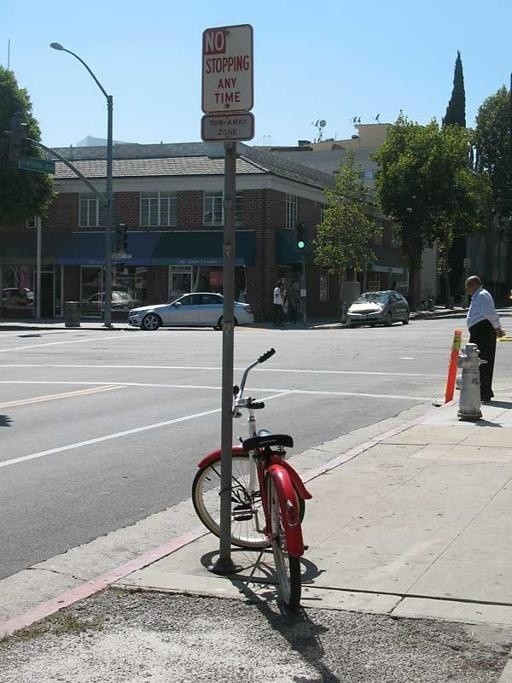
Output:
<box><xmin>49</xmin><ymin>41</ymin><xmax>114</xmax><ymax>328</ymax></box>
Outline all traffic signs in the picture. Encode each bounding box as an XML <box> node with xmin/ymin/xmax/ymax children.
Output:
<box><xmin>201</xmin><ymin>23</ymin><xmax>254</xmax><ymax>115</ymax></box>
<box><xmin>200</xmin><ymin>111</ymin><xmax>255</xmax><ymax>141</ymax></box>
<box><xmin>18</xmin><ymin>156</ymin><xmax>55</xmax><ymax>175</ymax></box>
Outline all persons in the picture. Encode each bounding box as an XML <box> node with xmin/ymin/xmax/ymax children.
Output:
<box><xmin>465</xmin><ymin>275</ymin><xmax>507</xmax><ymax>402</ymax></box>
<box><xmin>273</xmin><ymin>281</ymin><xmax>286</xmax><ymax>327</ymax></box>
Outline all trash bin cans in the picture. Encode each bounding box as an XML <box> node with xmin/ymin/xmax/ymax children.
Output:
<box><xmin>64</xmin><ymin>301</ymin><xmax>81</xmax><ymax>327</ymax></box>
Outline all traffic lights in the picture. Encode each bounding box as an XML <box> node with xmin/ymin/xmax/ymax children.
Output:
<box><xmin>14</xmin><ymin>122</ymin><xmax>33</xmax><ymax>148</ymax></box>
<box><xmin>117</xmin><ymin>222</ymin><xmax>128</xmax><ymax>248</ymax></box>
<box><xmin>297</xmin><ymin>223</ymin><xmax>306</xmax><ymax>249</ymax></box>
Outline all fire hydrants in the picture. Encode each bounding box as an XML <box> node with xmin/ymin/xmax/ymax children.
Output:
<box><xmin>457</xmin><ymin>343</ymin><xmax>489</xmax><ymax>420</ymax></box>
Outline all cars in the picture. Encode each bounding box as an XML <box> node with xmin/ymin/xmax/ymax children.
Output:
<box><xmin>0</xmin><ymin>287</ymin><xmax>34</xmax><ymax>316</ymax></box>
<box><xmin>346</xmin><ymin>289</ymin><xmax>410</xmax><ymax>328</ymax></box>
<box><xmin>128</xmin><ymin>292</ymin><xmax>255</xmax><ymax>330</ymax></box>
<box><xmin>82</xmin><ymin>290</ymin><xmax>136</xmax><ymax>310</ymax></box>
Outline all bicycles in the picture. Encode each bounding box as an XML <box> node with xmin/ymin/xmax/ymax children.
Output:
<box><xmin>191</xmin><ymin>345</ymin><xmax>312</xmax><ymax>613</ymax></box>
